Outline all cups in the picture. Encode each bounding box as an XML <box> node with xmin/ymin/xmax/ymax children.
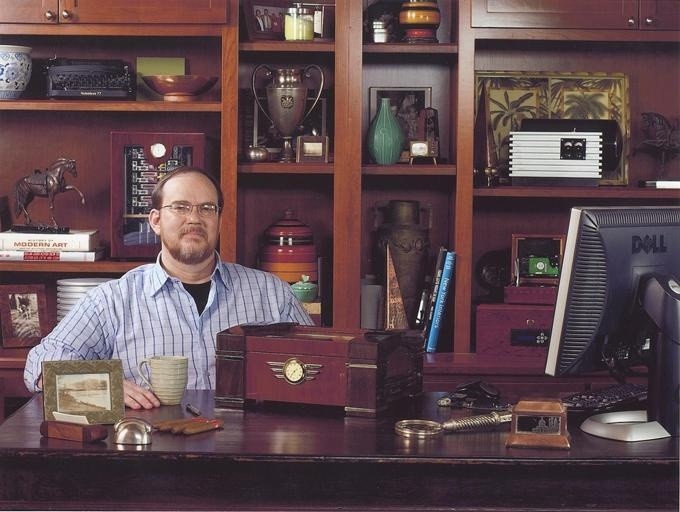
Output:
<box><xmin>138</xmin><ymin>355</ymin><xmax>188</xmax><ymax>406</ymax></box>
<box><xmin>292</xmin><ymin>273</ymin><xmax>318</xmax><ymax>303</ymax></box>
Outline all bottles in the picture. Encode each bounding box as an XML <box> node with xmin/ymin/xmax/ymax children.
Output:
<box><xmin>366</xmin><ymin>97</ymin><xmax>404</xmax><ymax>165</ymax></box>
<box><xmin>285</xmin><ymin>0</ymin><xmax>314</xmax><ymax>42</ymax></box>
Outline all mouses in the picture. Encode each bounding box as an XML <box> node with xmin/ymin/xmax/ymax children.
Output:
<box><xmin>456</xmin><ymin>381</ymin><xmax>502</xmax><ymax>403</ymax></box>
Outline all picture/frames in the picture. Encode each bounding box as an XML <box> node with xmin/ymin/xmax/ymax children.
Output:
<box><xmin>244</xmin><ymin>0</ymin><xmax>294</xmax><ymax>42</ymax></box>
<box><xmin>41</xmin><ymin>355</ymin><xmax>125</xmax><ymax>426</ymax></box>
<box><xmin>367</xmin><ymin>85</ymin><xmax>431</xmax><ymax>164</ymax></box>
<box><xmin>475</xmin><ymin>67</ymin><xmax>634</xmax><ymax>190</ymax></box>
<box><xmin>253</xmin><ymin>95</ymin><xmax>329</xmax><ymax>164</ymax></box>
<box><xmin>0</xmin><ymin>282</ymin><xmax>50</xmax><ymax>351</ymax></box>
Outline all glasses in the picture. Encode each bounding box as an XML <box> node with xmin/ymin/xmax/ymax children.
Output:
<box><xmin>159</xmin><ymin>200</ymin><xmax>221</xmax><ymax>217</ymax></box>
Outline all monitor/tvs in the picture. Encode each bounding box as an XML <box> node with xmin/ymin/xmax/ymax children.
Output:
<box><xmin>544</xmin><ymin>206</ymin><xmax>679</xmax><ymax>442</ymax></box>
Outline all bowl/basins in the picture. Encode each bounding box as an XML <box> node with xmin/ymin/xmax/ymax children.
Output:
<box><xmin>141</xmin><ymin>73</ymin><xmax>218</xmax><ymax>102</ymax></box>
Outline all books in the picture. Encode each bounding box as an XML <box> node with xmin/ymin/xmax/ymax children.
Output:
<box><xmin>0</xmin><ymin>229</ymin><xmax>106</xmax><ymax>262</ymax></box>
<box><xmin>413</xmin><ymin>248</ymin><xmax>457</xmax><ymax>352</ymax></box>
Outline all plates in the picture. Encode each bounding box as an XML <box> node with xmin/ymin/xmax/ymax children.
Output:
<box><xmin>56</xmin><ymin>277</ymin><xmax>115</xmax><ymax>327</ymax></box>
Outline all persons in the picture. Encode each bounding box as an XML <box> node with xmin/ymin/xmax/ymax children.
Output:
<box><xmin>396</xmin><ymin>105</ymin><xmax>420</xmax><ymax>150</ymax></box>
<box><xmin>256</xmin><ymin>8</ymin><xmax>283</xmax><ymax>33</ymax></box>
<box><xmin>24</xmin><ymin>165</ymin><xmax>317</xmax><ymax>411</ymax></box>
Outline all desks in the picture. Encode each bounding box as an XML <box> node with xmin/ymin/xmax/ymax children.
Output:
<box><xmin>0</xmin><ymin>391</ymin><xmax>678</xmax><ymax>507</ymax></box>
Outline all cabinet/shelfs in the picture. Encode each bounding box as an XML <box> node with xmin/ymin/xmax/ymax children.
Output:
<box><xmin>1</xmin><ymin>0</ymin><xmax>679</xmax><ymax>398</ymax></box>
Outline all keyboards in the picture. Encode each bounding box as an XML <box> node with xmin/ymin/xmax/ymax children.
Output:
<box><xmin>561</xmin><ymin>380</ymin><xmax>649</xmax><ymax>414</ymax></box>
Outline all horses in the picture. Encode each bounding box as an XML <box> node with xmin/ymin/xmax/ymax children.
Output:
<box><xmin>14</xmin><ymin>157</ymin><xmax>87</xmax><ymax>229</ymax></box>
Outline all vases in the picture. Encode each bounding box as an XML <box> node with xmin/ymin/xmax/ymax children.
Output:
<box><xmin>365</xmin><ymin>96</ymin><xmax>408</xmax><ymax>166</ymax></box>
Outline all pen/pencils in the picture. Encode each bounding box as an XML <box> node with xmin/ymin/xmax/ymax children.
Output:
<box><xmin>186</xmin><ymin>404</ymin><xmax>204</xmax><ymax>416</ymax></box>
